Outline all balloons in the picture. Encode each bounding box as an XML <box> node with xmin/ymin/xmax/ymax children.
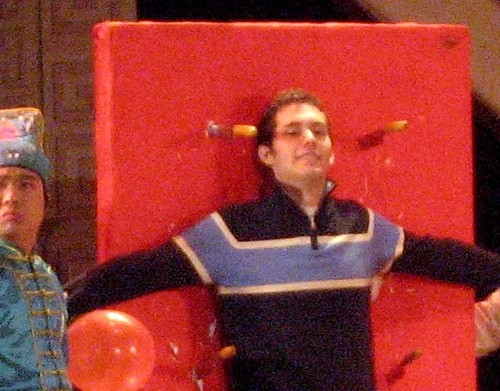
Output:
<box><xmin>66</xmin><ymin>308</ymin><xmax>155</xmax><ymax>391</ymax></box>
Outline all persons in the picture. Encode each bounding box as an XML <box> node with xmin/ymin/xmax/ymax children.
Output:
<box><xmin>62</xmin><ymin>89</ymin><xmax>500</xmax><ymax>391</ymax></box>
<box><xmin>0</xmin><ymin>107</ymin><xmax>72</xmax><ymax>391</ymax></box>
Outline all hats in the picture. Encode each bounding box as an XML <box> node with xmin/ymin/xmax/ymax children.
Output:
<box><xmin>0</xmin><ymin>107</ymin><xmax>56</xmax><ymax>183</ymax></box>
